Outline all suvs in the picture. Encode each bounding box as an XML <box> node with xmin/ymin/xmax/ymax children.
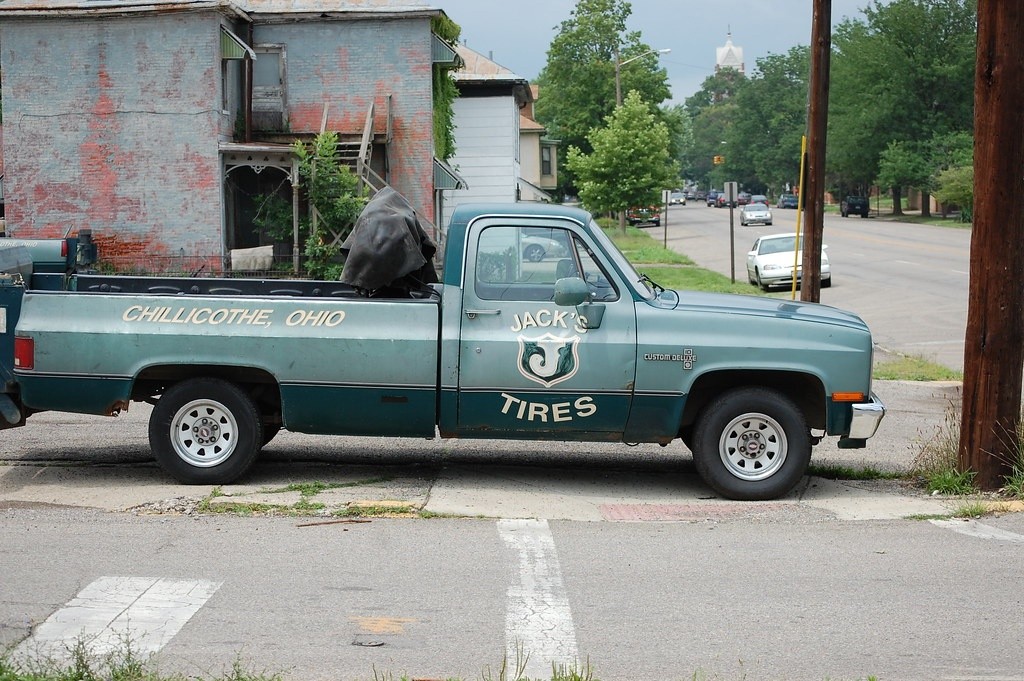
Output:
<box><xmin>841</xmin><ymin>196</ymin><xmax>870</xmax><ymax>218</ymax></box>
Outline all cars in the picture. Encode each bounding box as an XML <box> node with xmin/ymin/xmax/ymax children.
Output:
<box><xmin>626</xmin><ymin>204</ymin><xmax>662</xmax><ymax>228</ymax></box>
<box><xmin>740</xmin><ymin>204</ymin><xmax>773</xmax><ymax>227</ymax></box>
<box><xmin>776</xmin><ymin>194</ymin><xmax>798</xmax><ymax>209</ymax></box>
<box><xmin>668</xmin><ymin>192</ymin><xmax>687</xmax><ymax>206</ymax></box>
<box><xmin>746</xmin><ymin>232</ymin><xmax>832</xmax><ymax>293</ymax></box>
<box><xmin>522</xmin><ymin>226</ymin><xmax>570</xmax><ymax>262</ymax></box>
<box><xmin>681</xmin><ymin>189</ymin><xmax>770</xmax><ymax>208</ymax></box>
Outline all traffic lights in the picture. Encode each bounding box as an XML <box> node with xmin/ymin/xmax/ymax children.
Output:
<box><xmin>713</xmin><ymin>156</ymin><xmax>725</xmax><ymax>165</ymax></box>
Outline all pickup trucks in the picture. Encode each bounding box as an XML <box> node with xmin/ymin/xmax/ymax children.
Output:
<box><xmin>0</xmin><ymin>226</ymin><xmax>99</xmax><ymax>286</ymax></box>
<box><xmin>10</xmin><ymin>199</ymin><xmax>887</xmax><ymax>503</ymax></box>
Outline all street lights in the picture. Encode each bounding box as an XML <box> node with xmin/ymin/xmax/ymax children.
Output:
<box><xmin>613</xmin><ymin>46</ymin><xmax>674</xmax><ymax>233</ymax></box>
